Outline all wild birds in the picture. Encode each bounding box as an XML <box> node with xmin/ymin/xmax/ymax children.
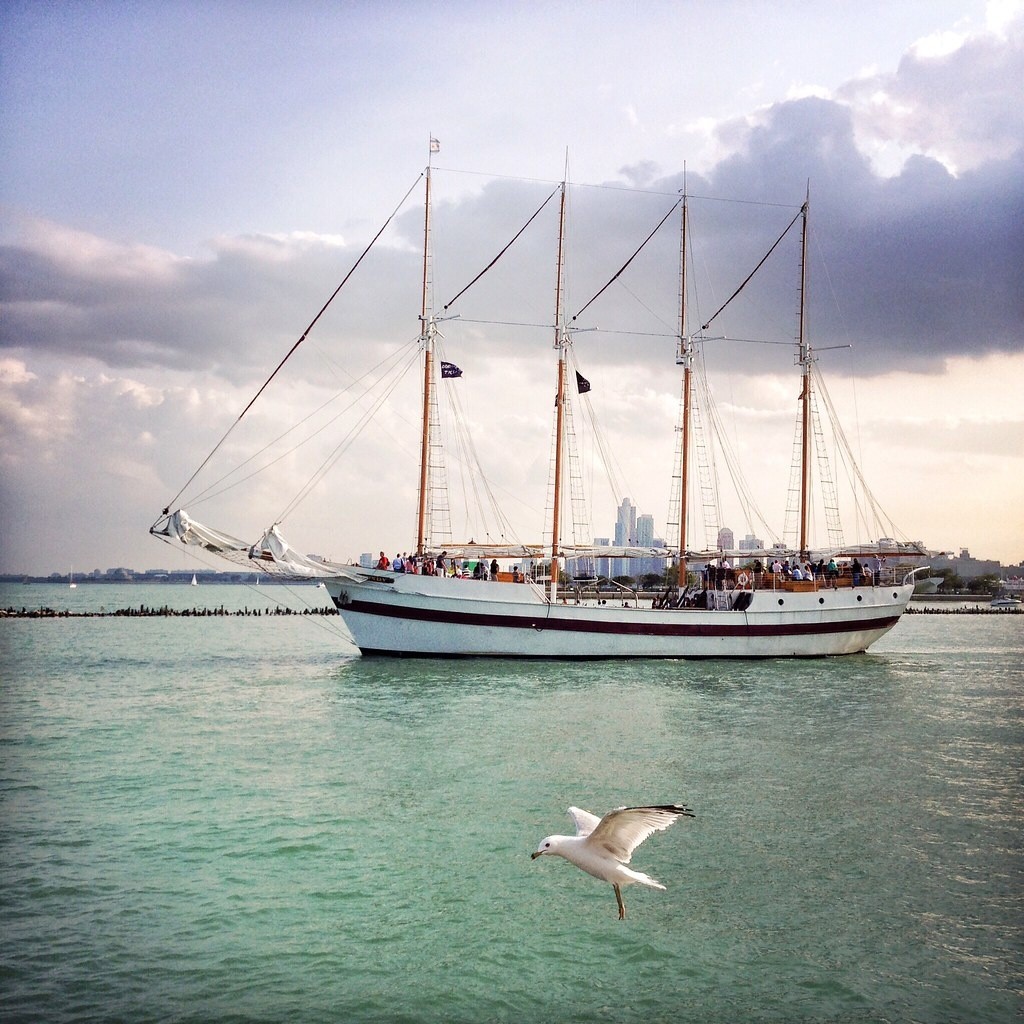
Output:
<box><xmin>532</xmin><ymin>803</ymin><xmax>695</xmax><ymax>921</ymax></box>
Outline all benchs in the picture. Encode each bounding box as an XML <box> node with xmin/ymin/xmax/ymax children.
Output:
<box><xmin>762</xmin><ymin>573</ymin><xmax>850</xmax><ymax>592</ymax></box>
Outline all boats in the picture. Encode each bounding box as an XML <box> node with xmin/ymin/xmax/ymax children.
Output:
<box><xmin>316</xmin><ymin>584</ymin><xmax>325</xmax><ymax>588</ymax></box>
<box><xmin>990</xmin><ymin>598</ymin><xmax>1022</xmax><ymax>608</ymax></box>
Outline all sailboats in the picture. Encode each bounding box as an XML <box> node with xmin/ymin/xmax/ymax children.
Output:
<box><xmin>145</xmin><ymin>131</ymin><xmax>930</xmax><ymax>664</ymax></box>
<box><xmin>191</xmin><ymin>573</ymin><xmax>197</xmax><ymax>586</ymax></box>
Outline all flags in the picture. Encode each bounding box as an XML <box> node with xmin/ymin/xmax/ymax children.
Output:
<box><xmin>441</xmin><ymin>362</ymin><xmax>463</xmax><ymax>378</ymax></box>
<box><xmin>575</xmin><ymin>371</ymin><xmax>592</xmax><ymax>395</ymax></box>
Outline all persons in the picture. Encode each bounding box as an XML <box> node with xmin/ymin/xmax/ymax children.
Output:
<box><xmin>530</xmin><ymin>561</ymin><xmax>535</xmax><ymax>579</ymax></box>
<box><xmin>373</xmin><ymin>551</ymin><xmax>388</xmax><ymax>570</ymax></box>
<box><xmin>512</xmin><ymin>566</ymin><xmax>520</xmax><ymax>583</ymax></box>
<box><xmin>562</xmin><ymin>553</ymin><xmax>887</xmax><ymax>609</ymax></box>
<box><xmin>393</xmin><ymin>551</ymin><xmax>500</xmax><ymax>581</ymax></box>
